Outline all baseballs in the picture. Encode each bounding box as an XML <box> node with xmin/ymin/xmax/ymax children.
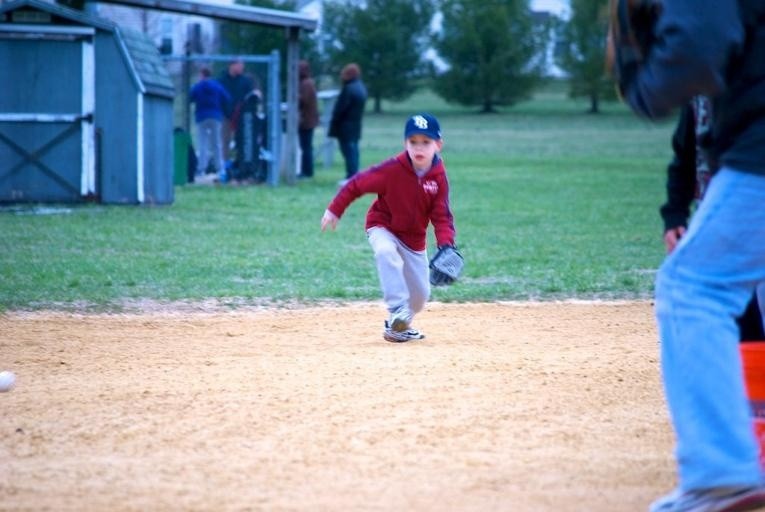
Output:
<box><xmin>1</xmin><ymin>371</ymin><xmax>15</xmax><ymax>391</ymax></box>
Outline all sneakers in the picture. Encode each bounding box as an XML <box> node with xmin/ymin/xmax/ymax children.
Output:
<box><xmin>648</xmin><ymin>483</ymin><xmax>765</xmax><ymax>512</ymax></box>
<box><xmin>390</xmin><ymin>305</ymin><xmax>412</xmax><ymax>331</ymax></box>
<box><xmin>382</xmin><ymin>319</ymin><xmax>427</xmax><ymax>343</ymax></box>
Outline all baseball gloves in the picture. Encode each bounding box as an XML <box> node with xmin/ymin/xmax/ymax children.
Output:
<box><xmin>431</xmin><ymin>246</ymin><xmax>462</xmax><ymax>286</ymax></box>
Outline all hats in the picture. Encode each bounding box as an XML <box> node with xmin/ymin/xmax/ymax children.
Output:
<box><xmin>405</xmin><ymin>112</ymin><xmax>441</xmax><ymax>140</ymax></box>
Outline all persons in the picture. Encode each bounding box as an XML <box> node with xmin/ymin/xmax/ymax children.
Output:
<box><xmin>320</xmin><ymin>111</ymin><xmax>464</xmax><ymax>343</ymax></box>
<box><xmin>660</xmin><ymin>91</ymin><xmax>765</xmax><ymax>478</ymax></box>
<box><xmin>602</xmin><ymin>1</ymin><xmax>765</xmax><ymax>511</ymax></box>
<box><xmin>187</xmin><ymin>60</ymin><xmax>367</xmax><ymax>185</ymax></box>
